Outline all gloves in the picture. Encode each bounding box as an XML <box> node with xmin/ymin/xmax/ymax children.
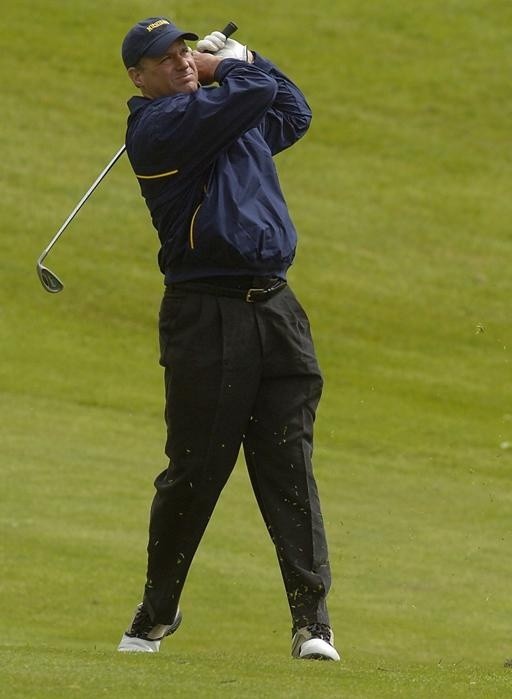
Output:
<box><xmin>188</xmin><ymin>29</ymin><xmax>248</xmax><ymax>62</ymax></box>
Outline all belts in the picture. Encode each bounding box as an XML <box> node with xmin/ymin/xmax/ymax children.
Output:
<box><xmin>173</xmin><ymin>280</ymin><xmax>287</xmax><ymax>302</ymax></box>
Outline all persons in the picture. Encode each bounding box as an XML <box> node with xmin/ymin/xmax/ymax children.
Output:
<box><xmin>116</xmin><ymin>16</ymin><xmax>341</xmax><ymax>664</ymax></box>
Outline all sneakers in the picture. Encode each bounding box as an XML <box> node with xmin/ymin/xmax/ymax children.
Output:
<box><xmin>116</xmin><ymin>601</ymin><xmax>183</xmax><ymax>652</ymax></box>
<box><xmin>290</xmin><ymin>623</ymin><xmax>341</xmax><ymax>662</ymax></box>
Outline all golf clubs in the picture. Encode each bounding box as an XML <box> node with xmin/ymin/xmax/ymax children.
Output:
<box><xmin>37</xmin><ymin>21</ymin><xmax>238</xmax><ymax>293</ymax></box>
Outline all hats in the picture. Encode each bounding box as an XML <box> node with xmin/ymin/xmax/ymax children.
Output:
<box><xmin>122</xmin><ymin>18</ymin><xmax>198</xmax><ymax>70</ymax></box>
